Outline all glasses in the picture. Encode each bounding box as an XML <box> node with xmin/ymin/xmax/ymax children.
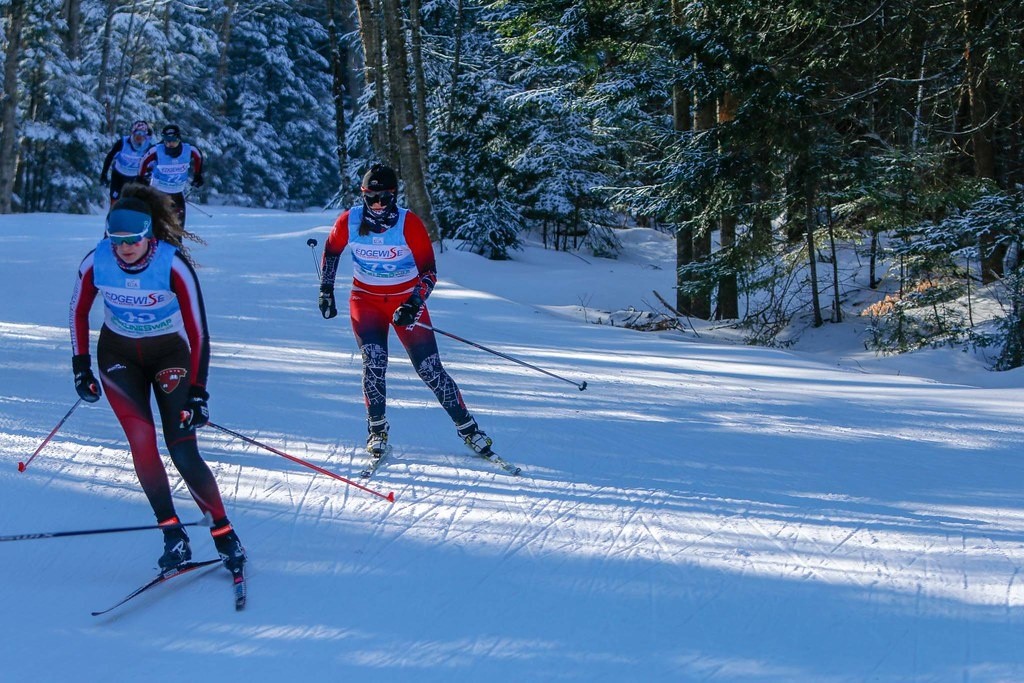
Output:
<box><xmin>364</xmin><ymin>191</ymin><xmax>395</xmax><ymax>206</ymax></box>
<box><xmin>134</xmin><ymin>130</ymin><xmax>146</xmax><ymax>136</ymax></box>
<box><xmin>106</xmin><ymin>229</ymin><xmax>149</xmax><ymax>246</ymax></box>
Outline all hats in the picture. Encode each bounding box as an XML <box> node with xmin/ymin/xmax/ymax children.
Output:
<box><xmin>361</xmin><ymin>164</ymin><xmax>397</xmax><ymax>192</ymax></box>
<box><xmin>162</xmin><ymin>124</ymin><xmax>180</xmax><ymax>136</ymax></box>
<box><xmin>132</xmin><ymin>123</ymin><xmax>148</xmax><ymax>133</ymax></box>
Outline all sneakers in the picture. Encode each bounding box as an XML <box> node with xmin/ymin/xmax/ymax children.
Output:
<box><xmin>212</xmin><ymin>530</ymin><xmax>248</xmax><ymax>569</ymax></box>
<box><xmin>156</xmin><ymin>524</ymin><xmax>192</xmax><ymax>566</ymax></box>
<box><xmin>456</xmin><ymin>414</ymin><xmax>492</xmax><ymax>453</ymax></box>
<box><xmin>365</xmin><ymin>415</ymin><xmax>389</xmax><ymax>453</ymax></box>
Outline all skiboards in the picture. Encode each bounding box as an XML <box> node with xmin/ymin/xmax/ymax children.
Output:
<box><xmin>361</xmin><ymin>444</ymin><xmax>522</xmax><ymax>480</ymax></box>
<box><xmin>91</xmin><ymin>556</ymin><xmax>247</xmax><ymax>616</ymax></box>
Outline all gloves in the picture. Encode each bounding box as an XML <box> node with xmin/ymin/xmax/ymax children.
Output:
<box><xmin>318</xmin><ymin>284</ymin><xmax>337</xmax><ymax>319</ymax></box>
<box><xmin>190</xmin><ymin>172</ymin><xmax>204</xmax><ymax>188</ymax></box>
<box><xmin>72</xmin><ymin>355</ymin><xmax>102</xmax><ymax>403</ymax></box>
<box><xmin>392</xmin><ymin>295</ymin><xmax>422</xmax><ymax>327</ymax></box>
<box><xmin>99</xmin><ymin>174</ymin><xmax>109</xmax><ymax>184</ymax></box>
<box><xmin>179</xmin><ymin>385</ymin><xmax>209</xmax><ymax>432</ymax></box>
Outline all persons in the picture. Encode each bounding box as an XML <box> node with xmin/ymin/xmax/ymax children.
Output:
<box><xmin>70</xmin><ymin>184</ymin><xmax>247</xmax><ymax>582</ymax></box>
<box><xmin>319</xmin><ymin>165</ymin><xmax>494</xmax><ymax>457</ymax></box>
<box><xmin>99</xmin><ymin>121</ymin><xmax>156</xmax><ymax>206</ymax></box>
<box><xmin>137</xmin><ymin>124</ymin><xmax>203</xmax><ymax>247</ymax></box>
